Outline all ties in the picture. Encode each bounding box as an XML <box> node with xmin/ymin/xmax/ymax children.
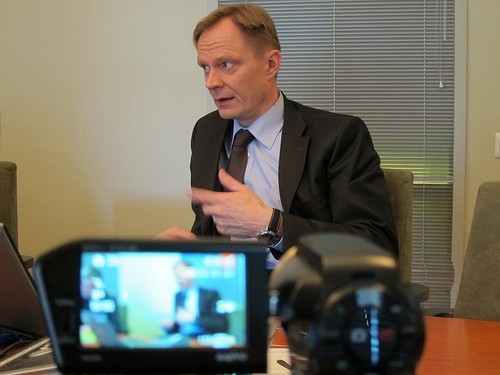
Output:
<box><xmin>225</xmin><ymin>130</ymin><xmax>255</xmax><ymax>187</ymax></box>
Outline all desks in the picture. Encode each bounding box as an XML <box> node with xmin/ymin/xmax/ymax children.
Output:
<box><xmin>272</xmin><ymin>315</ymin><xmax>500</xmax><ymax>375</ymax></box>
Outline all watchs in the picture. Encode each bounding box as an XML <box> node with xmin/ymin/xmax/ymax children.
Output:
<box><xmin>257</xmin><ymin>208</ymin><xmax>282</xmax><ymax>247</ymax></box>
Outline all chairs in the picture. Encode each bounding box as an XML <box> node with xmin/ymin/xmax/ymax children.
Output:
<box><xmin>421</xmin><ymin>181</ymin><xmax>500</xmax><ymax>322</ymax></box>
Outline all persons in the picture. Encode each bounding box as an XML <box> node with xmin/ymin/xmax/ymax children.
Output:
<box><xmin>150</xmin><ymin>4</ymin><xmax>398</xmax><ymax>276</ymax></box>
<box><xmin>158</xmin><ymin>260</ymin><xmax>229</xmax><ymax>339</ymax></box>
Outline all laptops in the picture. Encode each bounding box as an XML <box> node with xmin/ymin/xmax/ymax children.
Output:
<box><xmin>0</xmin><ymin>221</ymin><xmax>50</xmax><ymax>340</ymax></box>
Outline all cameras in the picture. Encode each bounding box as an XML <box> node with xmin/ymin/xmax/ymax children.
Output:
<box><xmin>34</xmin><ymin>232</ymin><xmax>426</xmax><ymax>374</ymax></box>
